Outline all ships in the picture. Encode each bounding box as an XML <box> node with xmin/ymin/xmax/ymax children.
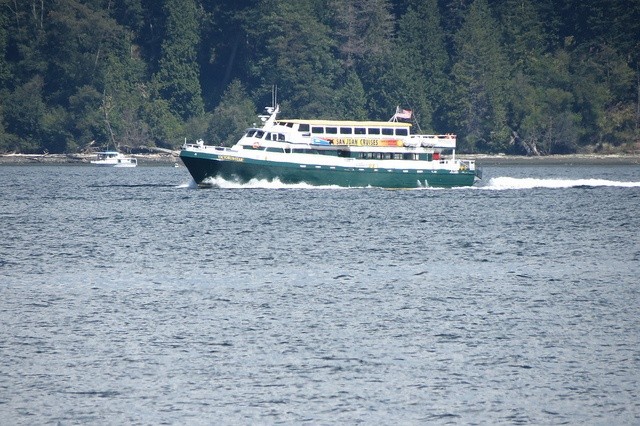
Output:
<box><xmin>178</xmin><ymin>104</ymin><xmax>482</xmax><ymax>188</ymax></box>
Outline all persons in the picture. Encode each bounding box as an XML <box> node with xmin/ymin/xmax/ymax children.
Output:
<box><xmin>196</xmin><ymin>138</ymin><xmax>204</xmax><ymax>149</ymax></box>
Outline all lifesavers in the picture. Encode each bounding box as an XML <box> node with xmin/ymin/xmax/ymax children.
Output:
<box><xmin>252</xmin><ymin>142</ymin><xmax>259</xmax><ymax>149</ymax></box>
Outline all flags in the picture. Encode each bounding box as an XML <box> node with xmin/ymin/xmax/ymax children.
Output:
<box><xmin>397</xmin><ymin>109</ymin><xmax>411</xmax><ymax>118</ymax></box>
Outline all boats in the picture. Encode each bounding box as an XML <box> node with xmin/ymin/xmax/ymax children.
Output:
<box><xmin>90</xmin><ymin>151</ymin><xmax>138</xmax><ymax>168</ymax></box>
